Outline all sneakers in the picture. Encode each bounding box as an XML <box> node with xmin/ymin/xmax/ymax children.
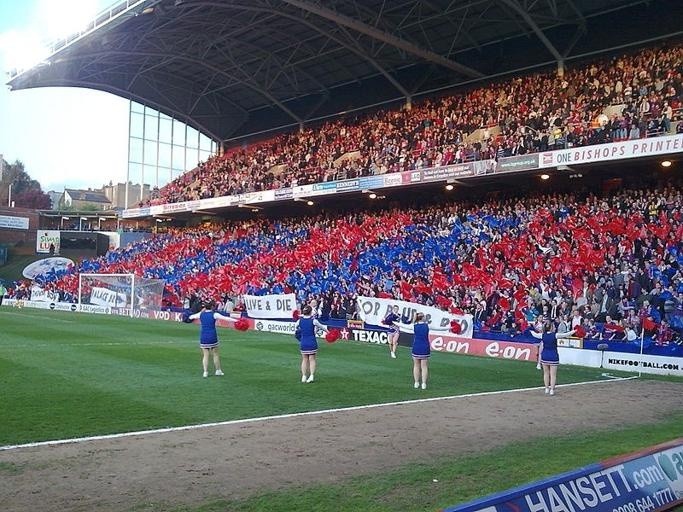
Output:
<box><xmin>391</xmin><ymin>351</ymin><xmax>397</xmax><ymax>359</ymax></box>
<box><xmin>414</xmin><ymin>382</ymin><xmax>427</xmax><ymax>389</ymax></box>
<box><xmin>545</xmin><ymin>388</ymin><xmax>554</xmax><ymax>396</ymax></box>
<box><xmin>204</xmin><ymin>369</ymin><xmax>225</xmax><ymax>377</ymax></box>
<box><xmin>536</xmin><ymin>363</ymin><xmax>541</xmax><ymax>370</ymax></box>
<box><xmin>301</xmin><ymin>374</ymin><xmax>315</xmax><ymax>383</ymax></box>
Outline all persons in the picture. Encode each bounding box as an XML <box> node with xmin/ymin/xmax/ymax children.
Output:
<box><xmin>294</xmin><ymin>306</ymin><xmax>341</xmax><ymax>384</ymax></box>
<box><xmin>185</xmin><ymin>302</ymin><xmax>248</xmax><ymax>377</ymax></box>
<box><xmin>525</xmin><ymin>323</ymin><xmax>585</xmax><ymax>395</ymax></box>
<box><xmin>382</xmin><ymin>306</ymin><xmax>406</xmax><ymax>359</ymax></box>
<box><xmin>391</xmin><ymin>313</ymin><xmax>456</xmax><ymax>390</ymax></box>
